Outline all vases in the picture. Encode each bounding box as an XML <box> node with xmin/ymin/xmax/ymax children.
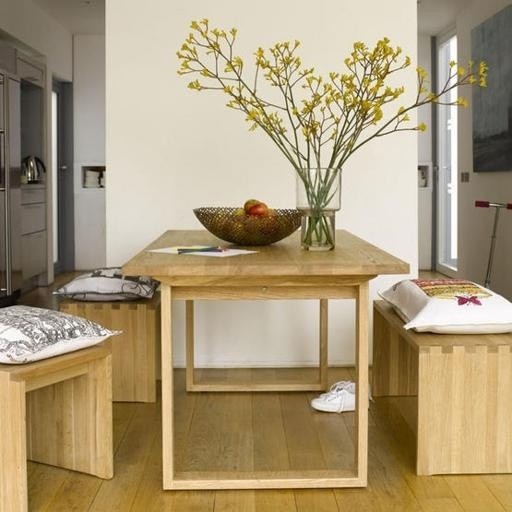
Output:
<box><xmin>296</xmin><ymin>168</ymin><xmax>342</xmax><ymax>252</ymax></box>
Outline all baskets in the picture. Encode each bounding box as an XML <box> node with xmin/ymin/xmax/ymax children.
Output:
<box><xmin>191</xmin><ymin>206</ymin><xmax>298</xmax><ymax>246</ymax></box>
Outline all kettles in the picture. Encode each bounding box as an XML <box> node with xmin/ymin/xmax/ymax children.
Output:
<box><xmin>24</xmin><ymin>155</ymin><xmax>47</xmax><ymax>184</ymax></box>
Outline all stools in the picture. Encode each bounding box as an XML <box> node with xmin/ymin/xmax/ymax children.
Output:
<box><xmin>0</xmin><ymin>338</ymin><xmax>116</xmax><ymax>508</ymax></box>
<box><xmin>57</xmin><ymin>291</ymin><xmax>160</xmax><ymax>403</ymax></box>
<box><xmin>371</xmin><ymin>300</ymin><xmax>511</xmax><ymax>476</ymax></box>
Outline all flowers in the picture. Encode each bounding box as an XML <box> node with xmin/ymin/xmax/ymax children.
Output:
<box><xmin>174</xmin><ymin>16</ymin><xmax>490</xmax><ymax>249</ymax></box>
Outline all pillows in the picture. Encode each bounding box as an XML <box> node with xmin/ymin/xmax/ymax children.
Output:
<box><xmin>375</xmin><ymin>270</ymin><xmax>511</xmax><ymax>335</ymax></box>
<box><xmin>57</xmin><ymin>258</ymin><xmax>156</xmax><ymax>303</ymax></box>
<box><xmin>1</xmin><ymin>303</ymin><xmax>128</xmax><ymax>365</ymax></box>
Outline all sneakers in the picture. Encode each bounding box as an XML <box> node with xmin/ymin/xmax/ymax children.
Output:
<box><xmin>311</xmin><ymin>381</ymin><xmax>373</xmax><ymax>413</ymax></box>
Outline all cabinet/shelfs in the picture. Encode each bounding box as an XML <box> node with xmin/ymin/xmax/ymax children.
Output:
<box><xmin>11</xmin><ymin>53</ymin><xmax>54</xmax><ymax>290</ymax></box>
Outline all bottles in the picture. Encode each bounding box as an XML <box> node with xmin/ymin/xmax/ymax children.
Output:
<box><xmin>21</xmin><ymin>163</ymin><xmax>28</xmax><ymax>183</ymax></box>
